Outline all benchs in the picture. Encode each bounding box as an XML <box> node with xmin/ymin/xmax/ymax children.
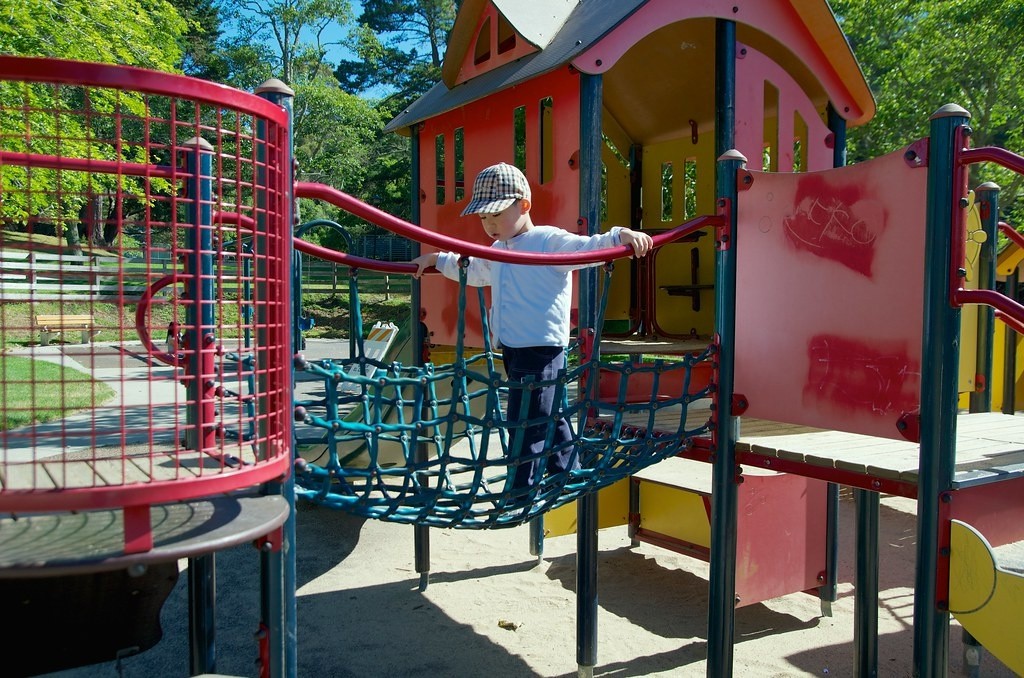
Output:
<box><xmin>34</xmin><ymin>313</ymin><xmax>107</xmax><ymax>346</ymax></box>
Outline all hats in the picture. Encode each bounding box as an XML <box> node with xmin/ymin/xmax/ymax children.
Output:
<box><xmin>458</xmin><ymin>161</ymin><xmax>531</xmax><ymax>218</ymax></box>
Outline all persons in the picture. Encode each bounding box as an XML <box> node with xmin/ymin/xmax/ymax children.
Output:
<box><xmin>405</xmin><ymin>161</ymin><xmax>652</xmax><ymax>520</ymax></box>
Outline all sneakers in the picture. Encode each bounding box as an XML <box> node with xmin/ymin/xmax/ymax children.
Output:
<box><xmin>496</xmin><ymin>495</ymin><xmax>546</xmax><ymax>525</ymax></box>
<box><xmin>541</xmin><ymin>475</ymin><xmax>585</xmax><ymax>495</ymax></box>
